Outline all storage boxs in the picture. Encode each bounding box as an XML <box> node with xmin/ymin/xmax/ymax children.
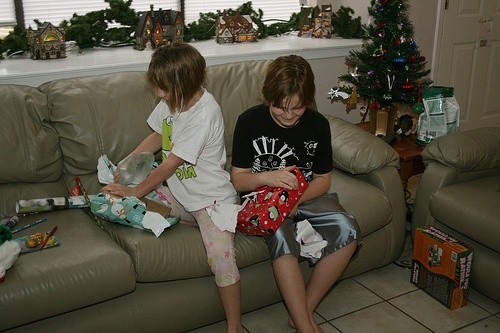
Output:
<box><xmin>101</xmin><ymin>190</ymin><xmax>172</xmax><ymax>219</ymax></box>
<box><xmin>237</xmin><ymin>168</ymin><xmax>309</xmax><ymax>236</ymax></box>
<box><xmin>410</xmin><ymin>225</ymin><xmax>474</xmax><ymax>311</ymax></box>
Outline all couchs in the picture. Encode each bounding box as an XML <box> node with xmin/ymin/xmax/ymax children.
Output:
<box><xmin>0</xmin><ymin>58</ymin><xmax>406</xmax><ymax>333</ymax></box>
<box><xmin>412</xmin><ymin>127</ymin><xmax>500</xmax><ymax>303</ymax></box>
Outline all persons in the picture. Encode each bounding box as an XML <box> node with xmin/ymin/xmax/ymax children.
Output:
<box><xmin>98</xmin><ymin>43</ymin><xmax>242</xmax><ymax>333</ymax></box>
<box><xmin>231</xmin><ymin>55</ymin><xmax>364</xmax><ymax>333</ymax></box>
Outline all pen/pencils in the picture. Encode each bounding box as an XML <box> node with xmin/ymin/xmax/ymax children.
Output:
<box><xmin>40</xmin><ymin>226</ymin><xmax>58</xmax><ymax>249</ymax></box>
<box><xmin>12</xmin><ymin>217</ymin><xmax>47</xmax><ymax>234</ymax></box>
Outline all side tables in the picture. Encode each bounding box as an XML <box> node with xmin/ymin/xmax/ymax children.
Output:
<box><xmin>355</xmin><ymin>118</ymin><xmax>425</xmax><ymax>189</ymax></box>
